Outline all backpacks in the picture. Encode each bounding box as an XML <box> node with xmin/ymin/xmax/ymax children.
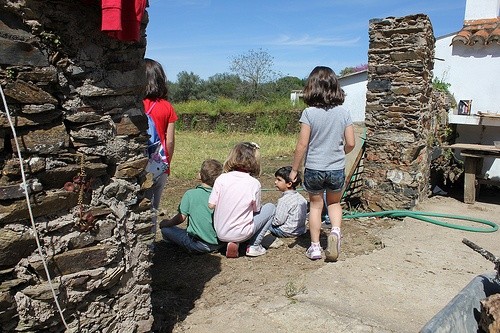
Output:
<box><xmin>143</xmin><ymin>98</ymin><xmax>169</xmax><ymax>182</ymax></box>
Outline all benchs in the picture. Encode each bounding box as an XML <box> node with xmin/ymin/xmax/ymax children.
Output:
<box><xmin>459</xmin><ymin>149</ymin><xmax>500</xmax><ymax>204</ymax></box>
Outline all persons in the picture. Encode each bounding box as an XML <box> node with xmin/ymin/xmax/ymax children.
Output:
<box><xmin>158</xmin><ymin>159</ymin><xmax>223</xmax><ymax>255</ymax></box>
<box><xmin>288</xmin><ymin>66</ymin><xmax>355</xmax><ymax>261</ymax></box>
<box><xmin>208</xmin><ymin>142</ymin><xmax>274</xmax><ymax>257</ymax></box>
<box><xmin>143</xmin><ymin>58</ymin><xmax>178</xmax><ymax>235</ymax></box>
<box><xmin>268</xmin><ymin>166</ymin><xmax>307</xmax><ymax>237</ymax></box>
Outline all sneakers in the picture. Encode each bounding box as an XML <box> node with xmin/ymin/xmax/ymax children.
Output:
<box><xmin>324</xmin><ymin>232</ymin><xmax>339</xmax><ymax>262</ymax></box>
<box><xmin>227</xmin><ymin>241</ymin><xmax>239</xmax><ymax>258</ymax></box>
<box><xmin>305</xmin><ymin>245</ymin><xmax>322</xmax><ymax>260</ymax></box>
<box><xmin>247</xmin><ymin>245</ymin><xmax>266</xmax><ymax>257</ymax></box>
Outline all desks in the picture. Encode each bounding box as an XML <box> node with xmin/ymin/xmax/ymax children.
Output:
<box><xmin>449</xmin><ymin>108</ymin><xmax>500</xmax><ymax>126</ymax></box>
